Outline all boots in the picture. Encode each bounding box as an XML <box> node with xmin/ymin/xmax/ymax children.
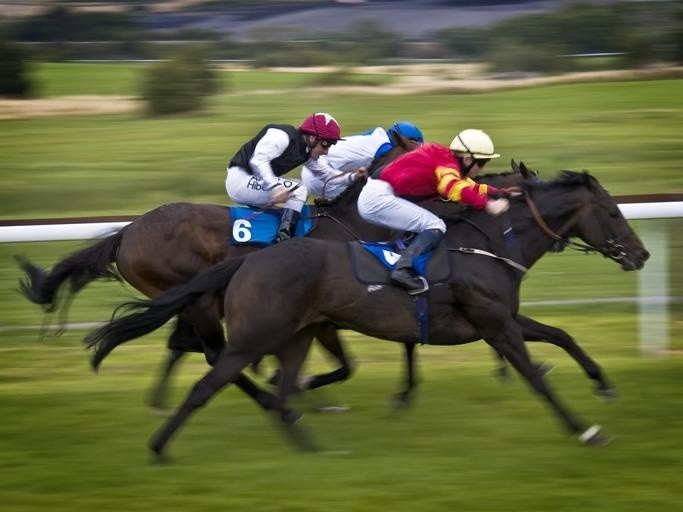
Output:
<box><xmin>391</xmin><ymin>229</ymin><xmax>444</xmax><ymax>288</ymax></box>
<box><xmin>276</xmin><ymin>208</ymin><xmax>300</xmax><ymax>243</ymax></box>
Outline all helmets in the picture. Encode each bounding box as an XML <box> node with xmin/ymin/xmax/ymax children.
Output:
<box><xmin>449</xmin><ymin>129</ymin><xmax>500</xmax><ymax>159</ymax></box>
<box><xmin>299</xmin><ymin>113</ymin><xmax>340</xmax><ymax>145</ymax></box>
<box><xmin>390</xmin><ymin>122</ymin><xmax>423</xmax><ymax>151</ymax></box>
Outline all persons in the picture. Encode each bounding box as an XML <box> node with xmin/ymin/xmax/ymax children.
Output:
<box><xmin>223</xmin><ymin>111</ymin><xmax>368</xmax><ymax>242</ymax></box>
<box><xmin>357</xmin><ymin>127</ymin><xmax>509</xmax><ymax>295</ymax></box>
<box><xmin>300</xmin><ymin>119</ymin><xmax>425</xmax><ymax>201</ymax></box>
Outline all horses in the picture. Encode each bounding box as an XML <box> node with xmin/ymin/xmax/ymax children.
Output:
<box><xmin>83</xmin><ymin>168</ymin><xmax>650</xmax><ymax>464</ymax></box>
<box><xmin>13</xmin><ymin>128</ymin><xmax>423</xmax><ymax>409</ymax></box>
<box><xmin>250</xmin><ymin>157</ymin><xmax>566</xmax><ymax>403</ymax></box>
<box><xmin>75</xmin><ymin>438</ymin><xmax>81</xmax><ymax>461</ymax></box>
